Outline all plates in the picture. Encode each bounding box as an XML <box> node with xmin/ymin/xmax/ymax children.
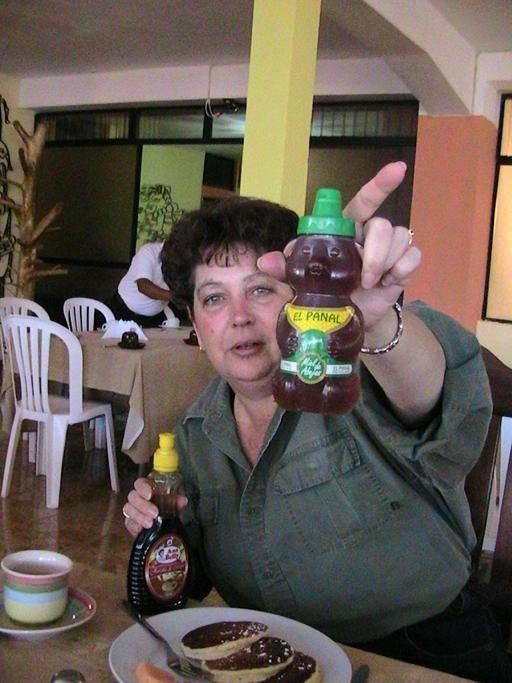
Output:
<box><xmin>108</xmin><ymin>606</ymin><xmax>354</xmax><ymax>683</ymax></box>
<box><xmin>0</xmin><ymin>586</ymin><xmax>98</xmax><ymax>641</ymax></box>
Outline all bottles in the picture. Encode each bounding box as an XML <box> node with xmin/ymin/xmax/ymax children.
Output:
<box><xmin>273</xmin><ymin>188</ymin><xmax>365</xmax><ymax>414</ymax></box>
<box><xmin>128</xmin><ymin>434</ymin><xmax>191</xmax><ymax>614</ymax></box>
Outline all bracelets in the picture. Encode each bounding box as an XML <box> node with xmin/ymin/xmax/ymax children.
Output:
<box><xmin>360</xmin><ymin>303</ymin><xmax>404</xmax><ymax>354</ymax></box>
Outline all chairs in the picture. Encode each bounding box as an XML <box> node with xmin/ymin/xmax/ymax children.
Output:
<box><xmin>0</xmin><ymin>297</ymin><xmax>119</xmax><ymax>510</ymax></box>
<box><xmin>478</xmin><ymin>349</ymin><xmax>512</xmax><ymax>578</ymax></box>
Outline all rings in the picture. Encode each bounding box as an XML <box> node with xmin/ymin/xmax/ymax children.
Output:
<box><xmin>408</xmin><ymin>229</ymin><xmax>415</xmax><ymax>246</ymax></box>
<box><xmin>123</xmin><ymin>505</ymin><xmax>130</xmax><ymax>518</ymax></box>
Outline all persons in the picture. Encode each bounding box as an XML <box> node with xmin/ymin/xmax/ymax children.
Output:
<box><xmin>122</xmin><ymin>161</ymin><xmax>512</xmax><ymax>683</ymax></box>
<box><xmin>94</xmin><ymin>238</ymin><xmax>177</xmax><ymax>331</ymax></box>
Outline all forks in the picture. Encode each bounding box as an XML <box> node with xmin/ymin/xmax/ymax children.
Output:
<box><xmin>116</xmin><ymin>598</ymin><xmax>204</xmax><ymax>681</ymax></box>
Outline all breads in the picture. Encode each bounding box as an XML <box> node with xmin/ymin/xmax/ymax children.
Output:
<box><xmin>181</xmin><ymin>621</ymin><xmax>322</xmax><ymax>683</ymax></box>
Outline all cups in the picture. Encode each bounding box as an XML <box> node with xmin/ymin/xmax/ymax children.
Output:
<box><xmin>1</xmin><ymin>548</ymin><xmax>72</xmax><ymax>623</ymax></box>
